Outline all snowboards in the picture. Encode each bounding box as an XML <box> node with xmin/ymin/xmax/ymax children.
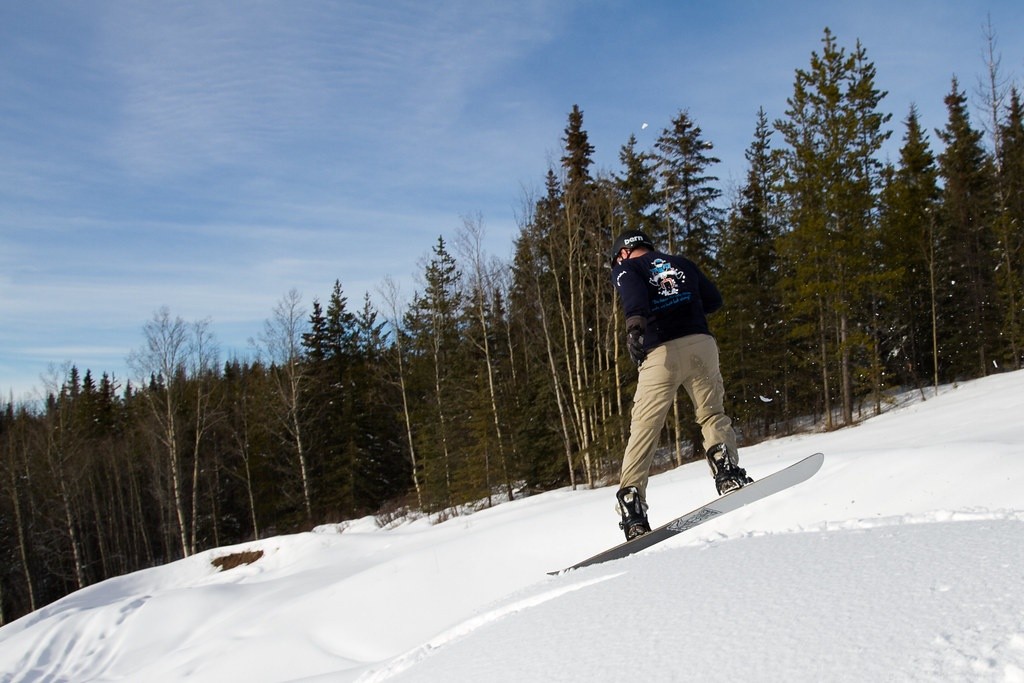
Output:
<box><xmin>546</xmin><ymin>451</ymin><xmax>824</xmax><ymax>575</ymax></box>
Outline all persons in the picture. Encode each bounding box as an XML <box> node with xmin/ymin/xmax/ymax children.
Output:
<box><xmin>612</xmin><ymin>230</ymin><xmax>754</xmax><ymax>542</ymax></box>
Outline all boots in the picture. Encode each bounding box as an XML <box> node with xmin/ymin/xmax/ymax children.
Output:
<box><xmin>706</xmin><ymin>442</ymin><xmax>754</xmax><ymax>494</ymax></box>
<box><xmin>615</xmin><ymin>486</ymin><xmax>648</xmax><ymax>540</ymax></box>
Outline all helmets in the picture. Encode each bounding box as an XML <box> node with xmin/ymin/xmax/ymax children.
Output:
<box><xmin>611</xmin><ymin>230</ymin><xmax>654</xmax><ymax>269</ymax></box>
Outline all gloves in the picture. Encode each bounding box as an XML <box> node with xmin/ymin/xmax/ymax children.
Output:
<box><xmin>625</xmin><ymin>316</ymin><xmax>647</xmax><ymax>365</ymax></box>
